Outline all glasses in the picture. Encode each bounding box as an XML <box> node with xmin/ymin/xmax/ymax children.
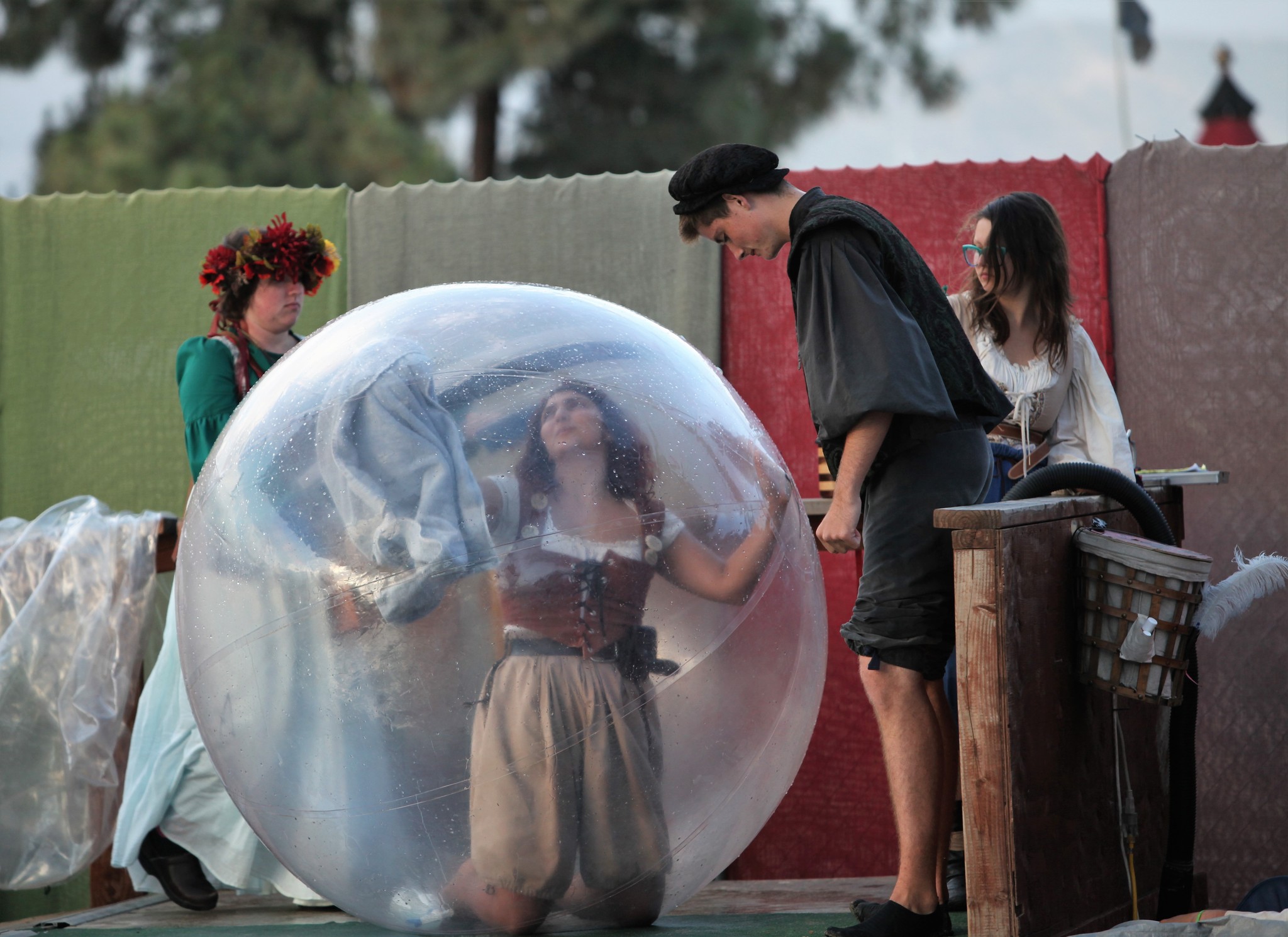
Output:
<box><xmin>962</xmin><ymin>244</ymin><xmax>1012</xmax><ymax>267</ymax></box>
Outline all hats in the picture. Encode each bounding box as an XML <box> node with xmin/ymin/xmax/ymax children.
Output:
<box><xmin>668</xmin><ymin>143</ymin><xmax>790</xmax><ymax>215</ymax></box>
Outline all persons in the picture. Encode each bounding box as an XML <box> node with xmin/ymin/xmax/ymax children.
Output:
<box><xmin>941</xmin><ymin>192</ymin><xmax>1139</xmax><ymax>911</ymax></box>
<box><xmin>669</xmin><ymin>140</ymin><xmax>998</xmax><ymax>937</ymax></box>
<box><xmin>136</xmin><ymin>216</ymin><xmax>380</xmax><ymax>933</ymax></box>
<box><xmin>441</xmin><ymin>379</ymin><xmax>792</xmax><ymax>937</ymax></box>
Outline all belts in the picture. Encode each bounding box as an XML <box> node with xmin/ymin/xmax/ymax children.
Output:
<box><xmin>989</xmin><ymin>423</ymin><xmax>1052</xmax><ymax>479</ymax></box>
<box><xmin>503</xmin><ymin>638</ymin><xmax>628</xmax><ymax>661</ymax></box>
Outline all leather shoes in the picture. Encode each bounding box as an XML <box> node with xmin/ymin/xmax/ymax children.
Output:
<box><xmin>297</xmin><ymin>905</ymin><xmax>343</xmax><ymax>912</ymax></box>
<box><xmin>137</xmin><ymin>827</ymin><xmax>218</xmax><ymax>910</ymax></box>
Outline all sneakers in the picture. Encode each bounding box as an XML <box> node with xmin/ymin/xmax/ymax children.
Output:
<box><xmin>944</xmin><ymin>848</ymin><xmax>967</xmax><ymax>910</ymax></box>
<box><xmin>823</xmin><ymin>897</ymin><xmax>950</xmax><ymax>937</ymax></box>
